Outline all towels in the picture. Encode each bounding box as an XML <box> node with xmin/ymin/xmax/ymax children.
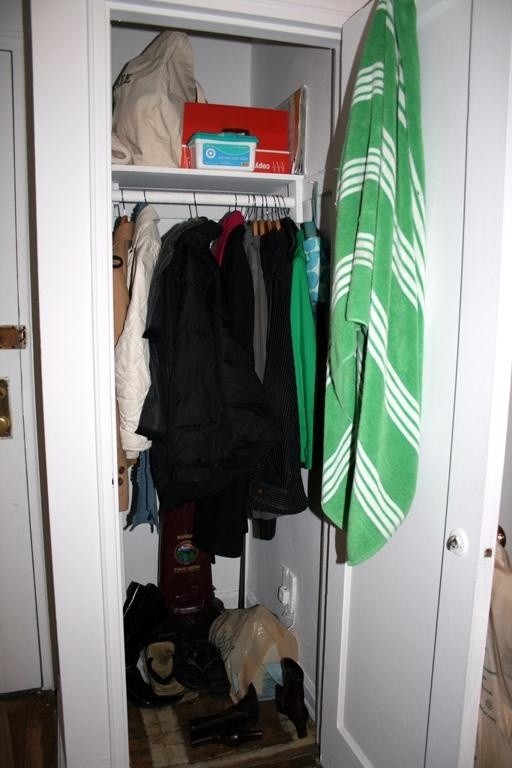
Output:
<box><xmin>300</xmin><ymin>1</ymin><xmax>424</xmax><ymax>567</ymax></box>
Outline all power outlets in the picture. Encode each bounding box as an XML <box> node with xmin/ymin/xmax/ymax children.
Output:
<box><xmin>277</xmin><ymin>565</ymin><xmax>296</xmax><ymax>615</ymax></box>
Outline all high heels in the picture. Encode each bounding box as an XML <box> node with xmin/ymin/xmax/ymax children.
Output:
<box><xmin>189</xmin><ymin>683</ymin><xmax>262</xmax><ymax>747</ymax></box>
<box><xmin>272</xmin><ymin>656</ymin><xmax>308</xmax><ymax>738</ymax></box>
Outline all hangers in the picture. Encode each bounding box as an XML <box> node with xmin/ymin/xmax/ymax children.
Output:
<box><xmin>112</xmin><ymin>185</ymin><xmax>293</xmax><ymax>242</ymax></box>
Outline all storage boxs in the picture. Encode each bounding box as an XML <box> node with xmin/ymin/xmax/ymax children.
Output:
<box><xmin>177</xmin><ymin>98</ymin><xmax>293</xmax><ymax>175</ymax></box>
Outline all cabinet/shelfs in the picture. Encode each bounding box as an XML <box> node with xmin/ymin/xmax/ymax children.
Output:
<box><xmin>26</xmin><ymin>1</ymin><xmax>508</xmax><ymax>767</ymax></box>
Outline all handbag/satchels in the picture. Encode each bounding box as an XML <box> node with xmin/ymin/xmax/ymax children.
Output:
<box><xmin>110</xmin><ymin>29</ymin><xmax>196</xmax><ymax>168</ymax></box>
